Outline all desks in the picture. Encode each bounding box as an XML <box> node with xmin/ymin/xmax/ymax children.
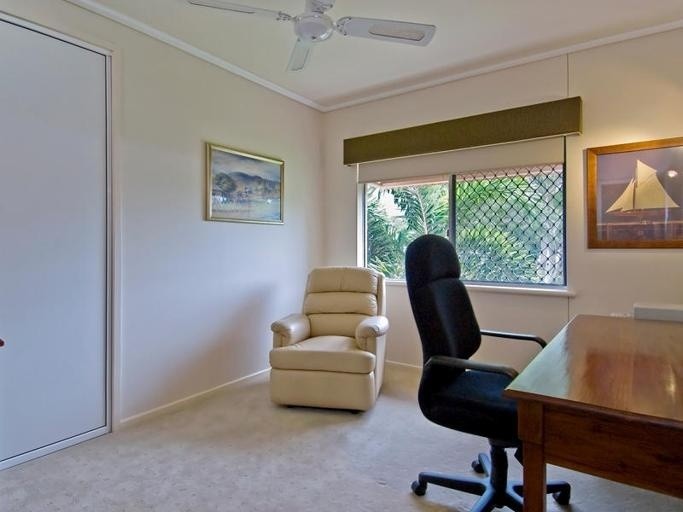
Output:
<box><xmin>501</xmin><ymin>311</ymin><xmax>682</xmax><ymax>512</ymax></box>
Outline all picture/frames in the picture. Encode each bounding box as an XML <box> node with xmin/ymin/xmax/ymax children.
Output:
<box><xmin>581</xmin><ymin>134</ymin><xmax>682</xmax><ymax>254</ymax></box>
<box><xmin>202</xmin><ymin>140</ymin><xmax>286</xmax><ymax>228</ymax></box>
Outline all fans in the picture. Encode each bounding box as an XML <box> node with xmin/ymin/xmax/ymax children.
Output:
<box><xmin>183</xmin><ymin>1</ymin><xmax>442</xmax><ymax>76</ymax></box>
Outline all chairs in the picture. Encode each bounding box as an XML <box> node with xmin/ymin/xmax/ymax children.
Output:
<box><xmin>402</xmin><ymin>229</ymin><xmax>574</xmax><ymax>512</ymax></box>
<box><xmin>264</xmin><ymin>262</ymin><xmax>388</xmax><ymax>415</ymax></box>
